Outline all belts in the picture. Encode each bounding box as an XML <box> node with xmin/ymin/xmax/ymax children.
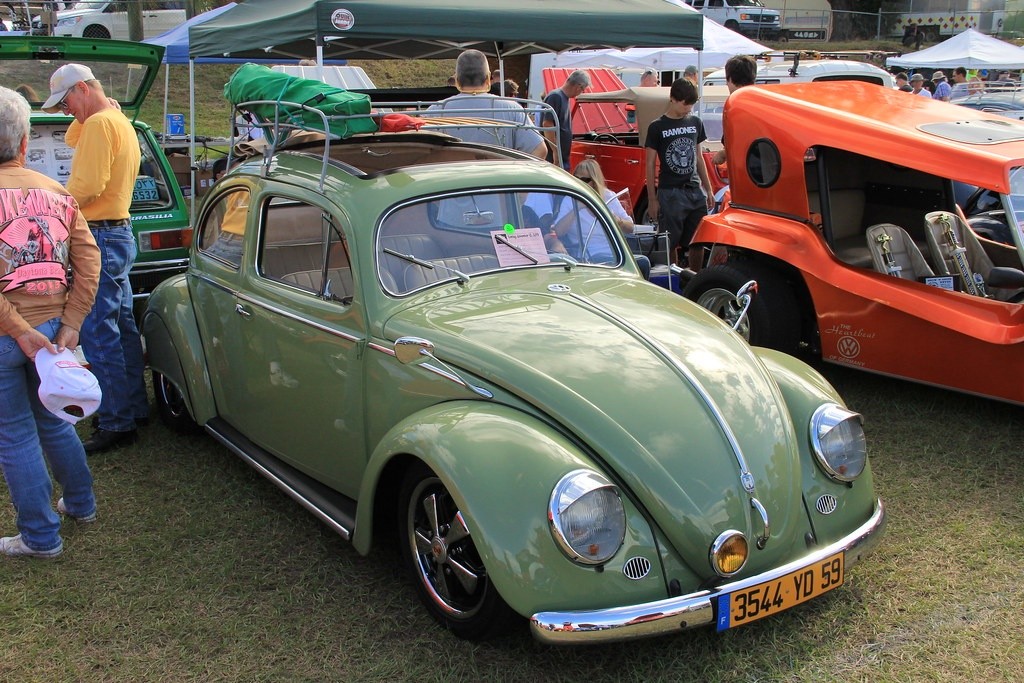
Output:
<box><xmin>88</xmin><ymin>219</ymin><xmax>130</xmax><ymax>228</ymax></box>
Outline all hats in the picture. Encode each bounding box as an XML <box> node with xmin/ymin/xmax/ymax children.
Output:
<box><xmin>35</xmin><ymin>344</ymin><xmax>102</xmax><ymax>425</ymax></box>
<box><xmin>41</xmin><ymin>63</ymin><xmax>95</xmax><ymax>114</ymax></box>
<box><xmin>931</xmin><ymin>71</ymin><xmax>945</xmax><ymax>81</ymax></box>
<box><xmin>911</xmin><ymin>74</ymin><xmax>924</xmax><ymax>81</ymax></box>
<box><xmin>684</xmin><ymin>65</ymin><xmax>698</xmax><ymax>74</ymax></box>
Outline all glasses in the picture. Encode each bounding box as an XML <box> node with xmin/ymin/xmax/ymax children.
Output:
<box><xmin>58</xmin><ymin>86</ymin><xmax>74</xmax><ymax>110</ymax></box>
<box><xmin>644</xmin><ymin>68</ymin><xmax>654</xmax><ymax>79</ymax></box>
<box><xmin>574</xmin><ymin>174</ymin><xmax>593</xmax><ymax>184</ymax></box>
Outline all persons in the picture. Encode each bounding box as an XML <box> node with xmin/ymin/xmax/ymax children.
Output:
<box><xmin>712</xmin><ymin>56</ymin><xmax>756</xmax><ymax>165</ymax></box>
<box><xmin>15</xmin><ymin>84</ymin><xmax>38</xmax><ymax>102</ymax></box>
<box><xmin>644</xmin><ymin>78</ymin><xmax>715</xmax><ymax>269</ymax></box>
<box><xmin>684</xmin><ymin>65</ymin><xmax>698</xmax><ymax>82</ymax></box>
<box><xmin>487</xmin><ymin>70</ymin><xmax>519</xmax><ymax>97</ymax></box>
<box><xmin>205</xmin><ymin>191</ymin><xmax>248</xmax><ymax>263</ymax></box>
<box><xmin>0</xmin><ymin>86</ymin><xmax>102</xmax><ymax>557</ymax></box>
<box><xmin>901</xmin><ymin>30</ymin><xmax>925</xmax><ymax>50</ymax></box>
<box><xmin>895</xmin><ymin>66</ymin><xmax>1017</xmax><ymax>101</ymax></box>
<box><xmin>212</xmin><ymin>159</ymin><xmax>233</xmax><ymax>183</ymax></box>
<box><xmin>641</xmin><ymin>70</ymin><xmax>658</xmax><ymax>87</ymax></box>
<box><xmin>448</xmin><ymin>76</ymin><xmax>455</xmax><ymax>86</ymax></box>
<box><xmin>554</xmin><ymin>159</ymin><xmax>651</xmax><ymax>280</ymax></box>
<box><xmin>522</xmin><ymin>206</ymin><xmax>567</xmax><ymax>254</ymax></box>
<box><xmin>42</xmin><ymin>63</ymin><xmax>149</xmax><ymax>456</ymax></box>
<box><xmin>540</xmin><ymin>69</ymin><xmax>591</xmax><ymax>172</ymax></box>
<box><xmin>0</xmin><ymin>18</ymin><xmax>8</xmax><ymax>31</ymax></box>
<box><xmin>423</xmin><ymin>50</ymin><xmax>549</xmax><ymax>235</ymax></box>
<box><xmin>299</xmin><ymin>59</ymin><xmax>316</xmax><ymax>66</ymax></box>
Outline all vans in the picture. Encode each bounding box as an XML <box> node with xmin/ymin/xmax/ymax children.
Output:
<box><xmin>52</xmin><ymin>1</ymin><xmax>188</xmax><ymax>41</ymax></box>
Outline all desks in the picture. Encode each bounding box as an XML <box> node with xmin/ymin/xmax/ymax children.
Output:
<box><xmin>160</xmin><ymin>138</ymin><xmax>228</xmax><ymax>148</ymax></box>
<box><xmin>629</xmin><ymin>231</ymin><xmax>672</xmax><ymax>295</ymax></box>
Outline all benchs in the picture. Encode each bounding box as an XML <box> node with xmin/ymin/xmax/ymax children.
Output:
<box><xmin>808</xmin><ymin>188</ymin><xmax>932</xmax><ymax>273</ymax></box>
<box><xmin>262</xmin><ymin>233</ymin><xmax>446</xmax><ymax>296</ymax></box>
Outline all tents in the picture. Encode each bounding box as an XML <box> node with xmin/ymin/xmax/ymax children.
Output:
<box><xmin>886</xmin><ymin>28</ymin><xmax>1024</xmax><ymax>69</ymax></box>
<box><xmin>527</xmin><ymin>0</ymin><xmax>784</xmax><ymax>119</ymax></box>
<box><xmin>123</xmin><ymin>2</ymin><xmax>347</xmax><ymax>154</ymax></box>
<box><xmin>189</xmin><ymin>0</ymin><xmax>703</xmax><ymax>226</ymax></box>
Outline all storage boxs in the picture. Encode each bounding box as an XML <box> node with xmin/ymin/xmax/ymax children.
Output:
<box><xmin>166</xmin><ymin>156</ymin><xmax>214</xmax><ymax>197</ymax></box>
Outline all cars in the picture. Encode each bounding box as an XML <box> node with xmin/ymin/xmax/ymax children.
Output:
<box><xmin>141</xmin><ymin>98</ymin><xmax>889</xmax><ymax>650</ymax></box>
<box><xmin>236</xmin><ymin>66</ymin><xmax>394</xmax><ymax>142</ymax></box>
<box><xmin>541</xmin><ymin>48</ymin><xmax>1024</xmax><ymax>226</ymax></box>
<box><xmin>0</xmin><ymin>34</ymin><xmax>195</xmax><ymax>324</ymax></box>
<box><xmin>678</xmin><ymin>79</ymin><xmax>1024</xmax><ymax>409</ymax></box>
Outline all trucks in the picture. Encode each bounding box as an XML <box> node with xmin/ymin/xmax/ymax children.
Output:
<box><xmin>881</xmin><ymin>0</ymin><xmax>1024</xmax><ymax>42</ymax></box>
<box><xmin>760</xmin><ymin>0</ymin><xmax>834</xmax><ymax>41</ymax></box>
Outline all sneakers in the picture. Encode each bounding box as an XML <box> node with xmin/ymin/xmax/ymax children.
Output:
<box><xmin>0</xmin><ymin>531</ymin><xmax>63</xmax><ymax>558</ymax></box>
<box><xmin>58</xmin><ymin>498</ymin><xmax>97</xmax><ymax>522</ymax></box>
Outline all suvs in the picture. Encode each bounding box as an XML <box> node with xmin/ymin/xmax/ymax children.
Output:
<box><xmin>684</xmin><ymin>0</ymin><xmax>782</xmax><ymax>42</ymax></box>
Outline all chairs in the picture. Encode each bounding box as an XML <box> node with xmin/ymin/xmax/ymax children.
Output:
<box><xmin>924</xmin><ymin>210</ymin><xmax>1024</xmax><ymax>301</ymax></box>
<box><xmin>866</xmin><ymin>223</ymin><xmax>936</xmax><ymax>280</ymax></box>
<box><xmin>281</xmin><ymin>268</ymin><xmax>397</xmax><ymax>298</ymax></box>
<box><xmin>403</xmin><ymin>252</ymin><xmax>499</xmax><ymax>291</ymax></box>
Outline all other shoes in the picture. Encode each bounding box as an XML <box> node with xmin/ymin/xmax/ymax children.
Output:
<box><xmin>80</xmin><ymin>428</ymin><xmax>135</xmax><ymax>453</ymax></box>
<box><xmin>91</xmin><ymin>416</ymin><xmax>152</xmax><ymax>427</ymax></box>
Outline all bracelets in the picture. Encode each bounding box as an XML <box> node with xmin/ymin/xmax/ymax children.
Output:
<box><xmin>618</xmin><ymin>217</ymin><xmax>623</xmax><ymax>225</ymax></box>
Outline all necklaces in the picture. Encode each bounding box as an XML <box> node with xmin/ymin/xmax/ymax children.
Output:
<box><xmin>462</xmin><ymin>90</ymin><xmax>486</xmax><ymax>95</ymax></box>
